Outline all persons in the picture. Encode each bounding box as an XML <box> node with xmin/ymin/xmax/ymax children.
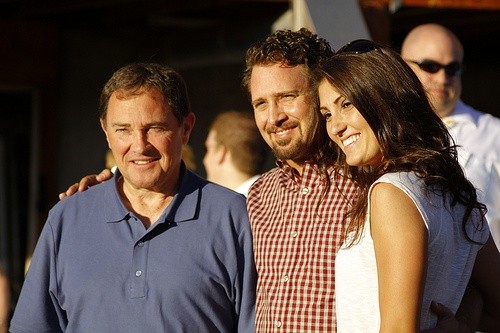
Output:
<box><xmin>8</xmin><ymin>62</ymin><xmax>258</xmax><ymax>333</ymax></box>
<box><xmin>399</xmin><ymin>23</ymin><xmax>500</xmax><ymax>252</ymax></box>
<box><xmin>202</xmin><ymin>110</ymin><xmax>265</xmax><ymax>199</ymax></box>
<box><xmin>320</xmin><ymin>39</ymin><xmax>500</xmax><ymax>333</ymax></box>
<box><xmin>59</xmin><ymin>26</ymin><xmax>487</xmax><ymax>333</ymax></box>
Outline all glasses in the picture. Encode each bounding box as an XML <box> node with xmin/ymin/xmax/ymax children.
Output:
<box><xmin>402</xmin><ymin>59</ymin><xmax>463</xmax><ymax>77</ymax></box>
<box><xmin>335</xmin><ymin>40</ymin><xmax>385</xmax><ymax>54</ymax></box>
